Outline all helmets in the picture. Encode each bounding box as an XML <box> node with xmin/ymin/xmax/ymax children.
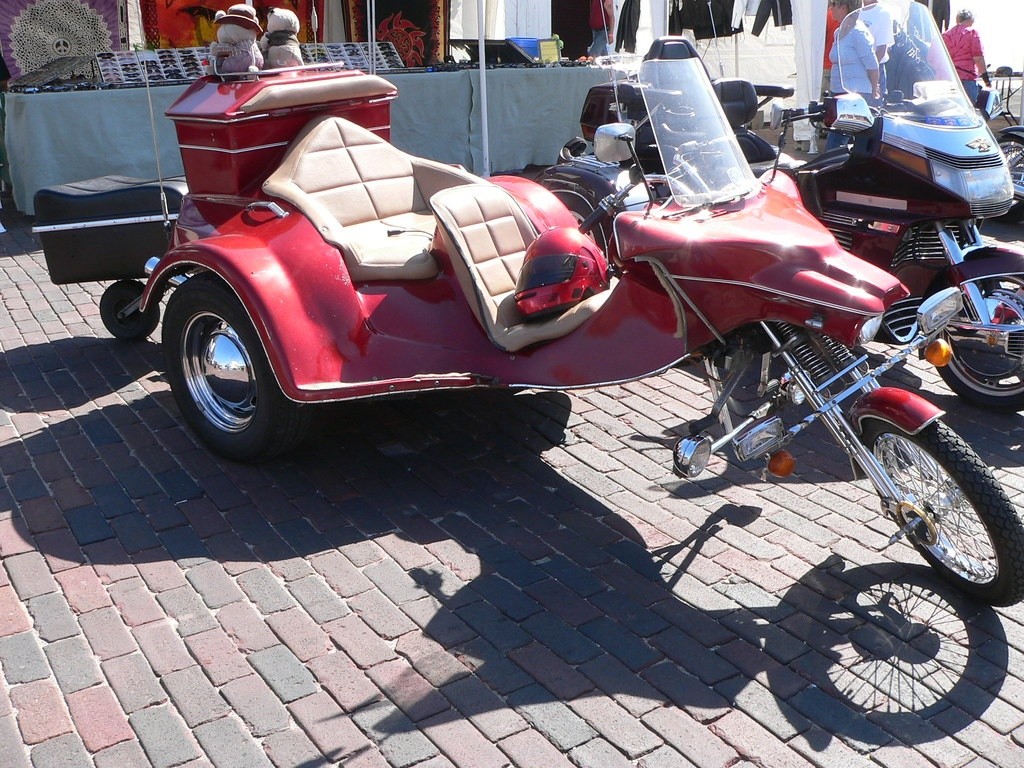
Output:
<box><xmin>514</xmin><ymin>227</ymin><xmax>611</xmax><ymax>320</ymax></box>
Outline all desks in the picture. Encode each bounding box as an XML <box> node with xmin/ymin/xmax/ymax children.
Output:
<box><xmin>977</xmin><ymin>77</ymin><xmax>1024</xmax><ymax>126</ymax></box>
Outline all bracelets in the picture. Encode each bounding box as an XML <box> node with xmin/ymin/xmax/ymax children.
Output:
<box><xmin>609</xmin><ymin>34</ymin><xmax>612</xmax><ymax>36</ymax></box>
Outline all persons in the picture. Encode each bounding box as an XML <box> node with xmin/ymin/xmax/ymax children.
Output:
<box><xmin>857</xmin><ymin>0</ymin><xmax>895</xmax><ymax>107</ymax></box>
<box><xmin>588</xmin><ymin>0</ymin><xmax>615</xmax><ymax>66</ymax></box>
<box><xmin>927</xmin><ymin>10</ymin><xmax>992</xmax><ymax>108</ymax></box>
<box><xmin>825</xmin><ymin>0</ymin><xmax>879</xmax><ymax>152</ymax></box>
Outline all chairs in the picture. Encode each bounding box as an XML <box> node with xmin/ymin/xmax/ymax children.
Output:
<box><xmin>712</xmin><ymin>78</ymin><xmax>777</xmax><ymax>164</ymax></box>
<box><xmin>263</xmin><ymin>115</ymin><xmax>620</xmax><ymax>353</ymax></box>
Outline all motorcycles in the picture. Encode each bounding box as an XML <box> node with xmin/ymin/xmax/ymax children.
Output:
<box><xmin>138</xmin><ymin>1</ymin><xmax>1023</xmax><ymax>609</ymax></box>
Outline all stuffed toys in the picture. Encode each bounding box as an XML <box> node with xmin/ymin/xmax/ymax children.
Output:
<box><xmin>205</xmin><ymin>4</ymin><xmax>304</xmax><ymax>77</ymax></box>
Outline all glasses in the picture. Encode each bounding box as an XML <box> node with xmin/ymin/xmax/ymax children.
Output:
<box><xmin>829</xmin><ymin>1</ymin><xmax>839</xmax><ymax>7</ymax></box>
